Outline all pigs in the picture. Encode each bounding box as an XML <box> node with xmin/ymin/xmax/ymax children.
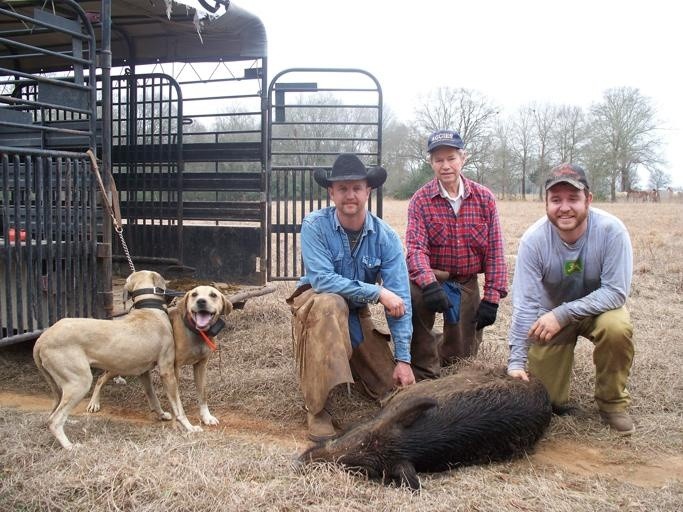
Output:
<box><xmin>294</xmin><ymin>362</ymin><xmax>554</xmax><ymax>496</ymax></box>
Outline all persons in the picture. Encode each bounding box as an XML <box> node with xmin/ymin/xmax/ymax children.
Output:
<box><xmin>505</xmin><ymin>160</ymin><xmax>636</xmax><ymax>434</ymax></box>
<box><xmin>281</xmin><ymin>152</ymin><xmax>421</xmax><ymax>441</ymax></box>
<box><xmin>403</xmin><ymin>130</ymin><xmax>513</xmax><ymax>382</ymax></box>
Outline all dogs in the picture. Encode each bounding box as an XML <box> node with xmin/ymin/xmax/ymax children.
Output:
<box><xmin>32</xmin><ymin>269</ymin><xmax>204</xmax><ymax>452</ymax></box>
<box><xmin>85</xmin><ymin>285</ymin><xmax>234</xmax><ymax>427</ymax></box>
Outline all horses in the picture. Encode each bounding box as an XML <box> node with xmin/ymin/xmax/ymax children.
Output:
<box><xmin>614</xmin><ymin>187</ymin><xmax>673</xmax><ymax>203</ymax></box>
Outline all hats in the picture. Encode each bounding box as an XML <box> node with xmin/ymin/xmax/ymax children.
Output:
<box><xmin>428</xmin><ymin>130</ymin><xmax>463</xmax><ymax>152</ymax></box>
<box><xmin>545</xmin><ymin>164</ymin><xmax>587</xmax><ymax>191</ymax></box>
<box><xmin>314</xmin><ymin>154</ymin><xmax>387</xmax><ymax>188</ymax></box>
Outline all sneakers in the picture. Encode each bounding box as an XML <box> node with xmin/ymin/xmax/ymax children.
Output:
<box><xmin>308</xmin><ymin>408</ymin><xmax>336</xmax><ymax>441</ymax></box>
<box><xmin>600</xmin><ymin>411</ymin><xmax>636</xmax><ymax>436</ymax></box>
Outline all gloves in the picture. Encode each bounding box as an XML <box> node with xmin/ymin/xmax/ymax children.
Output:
<box><xmin>422</xmin><ymin>281</ymin><xmax>452</xmax><ymax>313</ymax></box>
<box><xmin>472</xmin><ymin>300</ymin><xmax>498</xmax><ymax>331</ymax></box>
